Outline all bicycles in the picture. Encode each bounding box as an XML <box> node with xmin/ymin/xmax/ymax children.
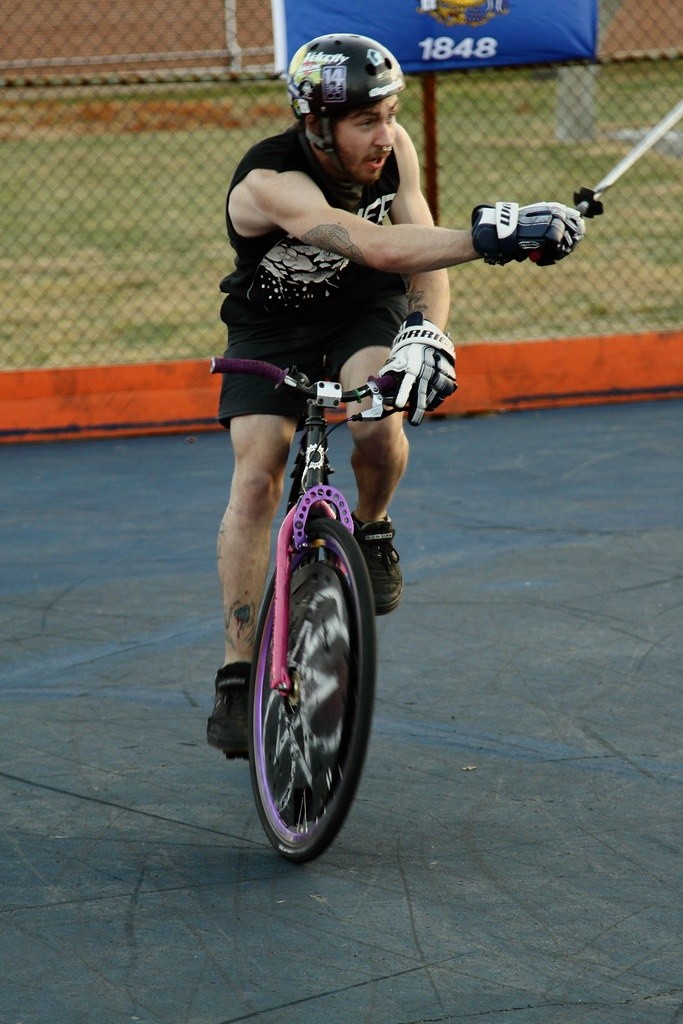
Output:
<box><xmin>203</xmin><ymin>350</ymin><xmax>416</xmax><ymax>868</ymax></box>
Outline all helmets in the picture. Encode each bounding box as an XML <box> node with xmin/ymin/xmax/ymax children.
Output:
<box><xmin>287</xmin><ymin>33</ymin><xmax>406</xmax><ymax>122</ymax></box>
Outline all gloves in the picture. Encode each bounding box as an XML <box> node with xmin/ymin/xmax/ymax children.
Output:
<box><xmin>470</xmin><ymin>200</ymin><xmax>585</xmax><ymax>266</ymax></box>
<box><xmin>378</xmin><ymin>311</ymin><xmax>460</xmax><ymax>424</ymax></box>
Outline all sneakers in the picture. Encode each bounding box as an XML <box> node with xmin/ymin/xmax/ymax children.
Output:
<box><xmin>206</xmin><ymin>669</ymin><xmax>251</xmax><ymax>752</ymax></box>
<box><xmin>353</xmin><ymin>513</ymin><xmax>404</xmax><ymax>614</ymax></box>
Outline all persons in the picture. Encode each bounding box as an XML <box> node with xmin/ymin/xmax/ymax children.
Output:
<box><xmin>203</xmin><ymin>33</ymin><xmax>587</xmax><ymax>752</ymax></box>
